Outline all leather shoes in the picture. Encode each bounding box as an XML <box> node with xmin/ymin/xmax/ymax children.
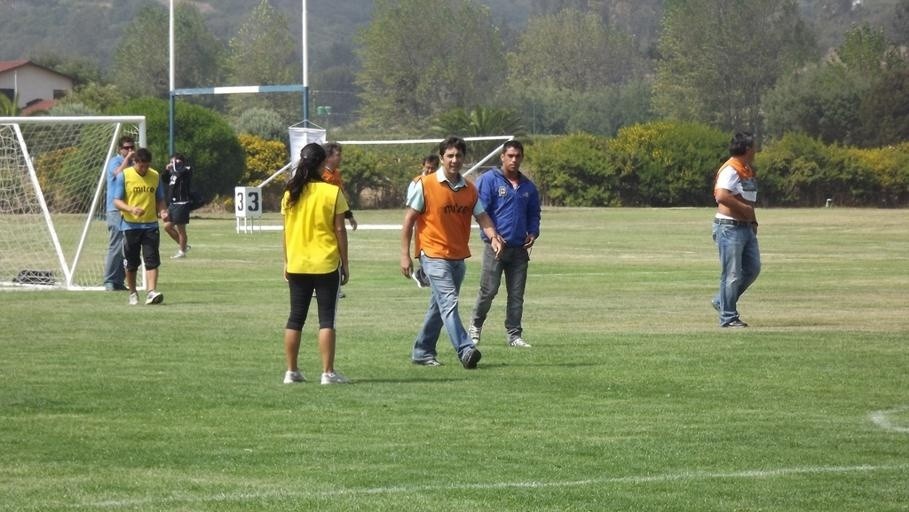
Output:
<box><xmin>723</xmin><ymin>318</ymin><xmax>747</xmax><ymax>327</ymax></box>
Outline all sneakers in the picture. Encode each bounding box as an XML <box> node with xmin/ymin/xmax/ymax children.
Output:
<box><xmin>510</xmin><ymin>336</ymin><xmax>532</xmax><ymax>347</ymax></box>
<box><xmin>130</xmin><ymin>291</ymin><xmax>140</xmax><ymax>305</ymax></box>
<box><xmin>145</xmin><ymin>290</ymin><xmax>164</xmax><ymax>305</ymax></box>
<box><xmin>461</xmin><ymin>347</ymin><xmax>482</xmax><ymax>368</ymax></box>
<box><xmin>320</xmin><ymin>371</ymin><xmax>350</xmax><ymax>385</ymax></box>
<box><xmin>468</xmin><ymin>325</ymin><xmax>483</xmax><ymax>345</ymax></box>
<box><xmin>169</xmin><ymin>251</ymin><xmax>187</xmax><ymax>259</ymax></box>
<box><xmin>411</xmin><ymin>269</ymin><xmax>427</xmax><ymax>288</ymax></box>
<box><xmin>283</xmin><ymin>369</ymin><xmax>308</xmax><ymax>384</ymax></box>
<box><xmin>410</xmin><ymin>357</ymin><xmax>441</xmax><ymax>367</ymax></box>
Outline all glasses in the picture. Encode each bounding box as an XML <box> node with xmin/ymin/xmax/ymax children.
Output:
<box><xmin>122</xmin><ymin>145</ymin><xmax>135</xmax><ymax>151</ymax></box>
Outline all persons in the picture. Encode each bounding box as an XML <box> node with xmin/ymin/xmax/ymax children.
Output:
<box><xmin>280</xmin><ymin>142</ymin><xmax>349</xmax><ymax>385</ymax></box>
<box><xmin>161</xmin><ymin>152</ymin><xmax>194</xmax><ymax>260</ymax></box>
<box><xmin>102</xmin><ymin>135</ymin><xmax>136</xmax><ymax>292</ymax></box>
<box><xmin>311</xmin><ymin>142</ymin><xmax>358</xmax><ymax>299</ymax></box>
<box><xmin>710</xmin><ymin>130</ymin><xmax>761</xmax><ymax>328</ymax></box>
<box><xmin>112</xmin><ymin>148</ymin><xmax>169</xmax><ymax>305</ymax></box>
<box><xmin>400</xmin><ymin>137</ymin><xmax>506</xmax><ymax>370</ymax></box>
<box><xmin>468</xmin><ymin>139</ymin><xmax>541</xmax><ymax>348</ymax></box>
<box><xmin>406</xmin><ymin>154</ymin><xmax>440</xmax><ymax>289</ymax></box>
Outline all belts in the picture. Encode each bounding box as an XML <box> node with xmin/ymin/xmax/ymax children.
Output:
<box><xmin>714</xmin><ymin>218</ymin><xmax>749</xmax><ymax>227</ymax></box>
